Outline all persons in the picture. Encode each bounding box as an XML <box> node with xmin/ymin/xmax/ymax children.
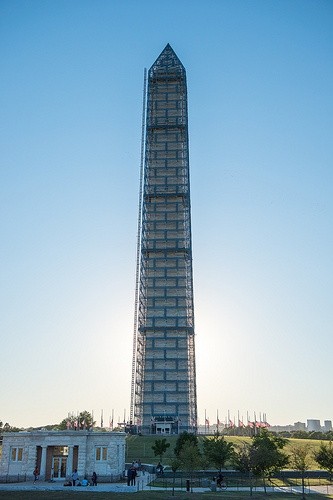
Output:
<box><xmin>157</xmin><ymin>462</ymin><xmax>164</xmax><ymax>475</ymax></box>
<box><xmin>33</xmin><ymin>467</ymin><xmax>39</xmax><ymax>484</ymax></box>
<box><xmin>72</xmin><ymin>469</ymin><xmax>78</xmax><ymax>486</ymax></box>
<box><xmin>132</xmin><ymin>459</ymin><xmax>141</xmax><ymax>477</ymax></box>
<box><xmin>127</xmin><ymin>466</ymin><xmax>136</xmax><ymax>486</ymax></box>
<box><xmin>75</xmin><ymin>478</ymin><xmax>92</xmax><ymax>486</ymax></box>
<box><xmin>92</xmin><ymin>471</ymin><xmax>97</xmax><ymax>486</ymax></box>
<box><xmin>214</xmin><ymin>472</ymin><xmax>224</xmax><ymax>486</ymax></box>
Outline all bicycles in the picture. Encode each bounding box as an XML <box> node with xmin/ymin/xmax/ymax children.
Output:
<box><xmin>208</xmin><ymin>477</ymin><xmax>228</xmax><ymax>491</ymax></box>
